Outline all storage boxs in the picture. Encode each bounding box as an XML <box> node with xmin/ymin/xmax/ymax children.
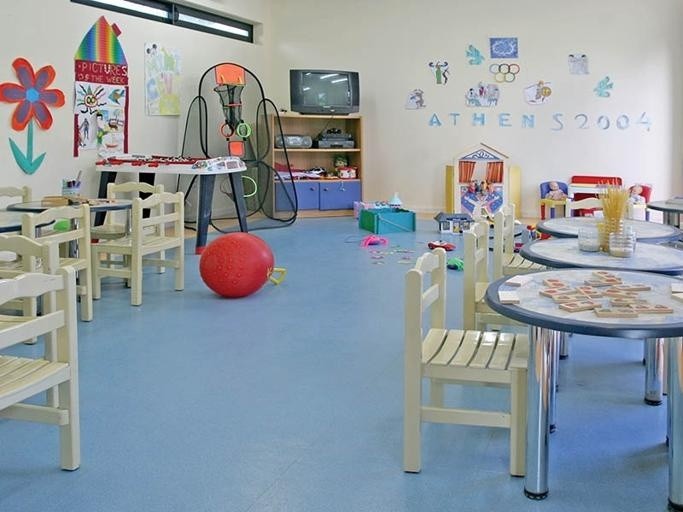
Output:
<box><xmin>352</xmin><ymin>200</ymin><xmax>402</xmax><ymax>219</ymax></box>
<box><xmin>357</xmin><ymin>206</ymin><xmax>416</xmax><ymax>235</ymax></box>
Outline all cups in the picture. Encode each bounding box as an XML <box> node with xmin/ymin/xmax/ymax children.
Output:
<box><xmin>608</xmin><ymin>232</ymin><xmax>636</xmax><ymax>257</ymax></box>
<box><xmin>625</xmin><ymin>225</ymin><xmax>632</xmax><ymax>233</ymax></box>
<box><xmin>577</xmin><ymin>227</ymin><xmax>601</xmax><ymax>251</ymax></box>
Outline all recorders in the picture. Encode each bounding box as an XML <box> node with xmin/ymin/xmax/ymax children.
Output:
<box><xmin>275</xmin><ymin>133</ymin><xmax>313</xmax><ymax>148</ymax></box>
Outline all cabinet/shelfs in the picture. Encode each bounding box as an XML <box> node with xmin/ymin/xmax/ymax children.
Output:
<box><xmin>256</xmin><ymin>110</ymin><xmax>364</xmax><ymax>220</ymax></box>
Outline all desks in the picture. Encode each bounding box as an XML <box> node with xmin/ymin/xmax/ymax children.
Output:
<box><xmin>91</xmin><ymin>152</ymin><xmax>251</xmax><ymax>255</ymax></box>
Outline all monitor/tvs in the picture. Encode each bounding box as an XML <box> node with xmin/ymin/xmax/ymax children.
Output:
<box><xmin>289</xmin><ymin>68</ymin><xmax>360</xmax><ymax>116</ymax></box>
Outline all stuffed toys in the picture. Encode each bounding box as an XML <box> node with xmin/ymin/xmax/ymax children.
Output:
<box><xmin>544</xmin><ymin>182</ymin><xmax>567</xmax><ymax>202</ymax></box>
<box><xmin>626</xmin><ymin>185</ymin><xmax>647</xmax><ymax>206</ymax></box>
<box><xmin>333</xmin><ymin>154</ymin><xmax>349</xmax><ymax>175</ymax></box>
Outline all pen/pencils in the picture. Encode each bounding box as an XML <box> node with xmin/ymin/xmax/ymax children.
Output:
<box><xmin>594</xmin><ymin>177</ymin><xmax>629</xmax><ymax>255</ymax></box>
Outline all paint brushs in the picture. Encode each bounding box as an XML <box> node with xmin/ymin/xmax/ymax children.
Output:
<box><xmin>63</xmin><ymin>171</ymin><xmax>82</xmax><ymax>188</ymax></box>
